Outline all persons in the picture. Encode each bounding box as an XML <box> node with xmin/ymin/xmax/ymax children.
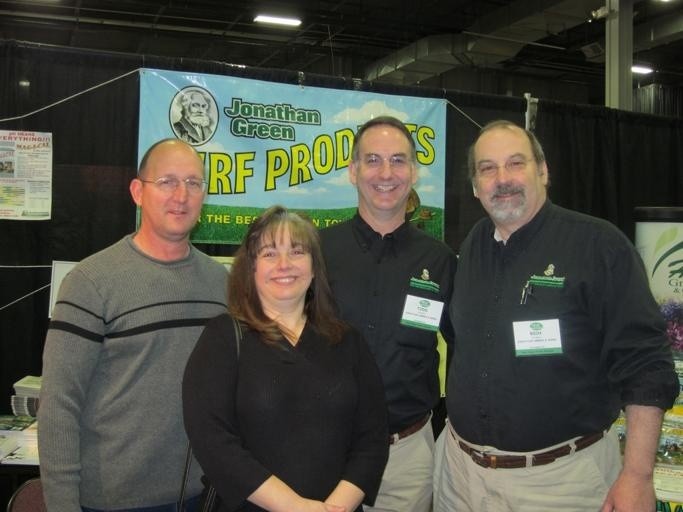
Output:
<box><xmin>172</xmin><ymin>90</ymin><xmax>214</xmax><ymax>143</ymax></box>
<box><xmin>34</xmin><ymin>137</ymin><xmax>235</xmax><ymax>510</ymax></box>
<box><xmin>181</xmin><ymin>205</ymin><xmax>391</xmax><ymax>510</ymax></box>
<box><xmin>433</xmin><ymin>118</ymin><xmax>680</xmax><ymax>511</ymax></box>
<box><xmin>304</xmin><ymin>114</ymin><xmax>459</xmax><ymax>512</ymax></box>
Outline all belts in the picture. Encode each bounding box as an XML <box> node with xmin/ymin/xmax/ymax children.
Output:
<box><xmin>449</xmin><ymin>428</ymin><xmax>610</xmax><ymax>469</ymax></box>
<box><xmin>389</xmin><ymin>411</ymin><xmax>431</xmax><ymax>443</ymax></box>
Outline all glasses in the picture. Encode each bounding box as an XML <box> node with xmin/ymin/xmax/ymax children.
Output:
<box><xmin>357</xmin><ymin>155</ymin><xmax>415</xmax><ymax>168</ymax></box>
<box><xmin>144</xmin><ymin>178</ymin><xmax>208</xmax><ymax>193</ymax></box>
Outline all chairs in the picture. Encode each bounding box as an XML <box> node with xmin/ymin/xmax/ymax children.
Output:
<box><xmin>7</xmin><ymin>479</ymin><xmax>48</xmax><ymax>512</ymax></box>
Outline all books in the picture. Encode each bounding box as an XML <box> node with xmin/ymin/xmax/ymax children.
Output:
<box><xmin>0</xmin><ymin>375</ymin><xmax>41</xmax><ymax>463</ymax></box>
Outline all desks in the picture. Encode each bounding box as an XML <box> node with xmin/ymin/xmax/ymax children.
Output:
<box><xmin>0</xmin><ymin>414</ymin><xmax>40</xmax><ymax>511</ymax></box>
